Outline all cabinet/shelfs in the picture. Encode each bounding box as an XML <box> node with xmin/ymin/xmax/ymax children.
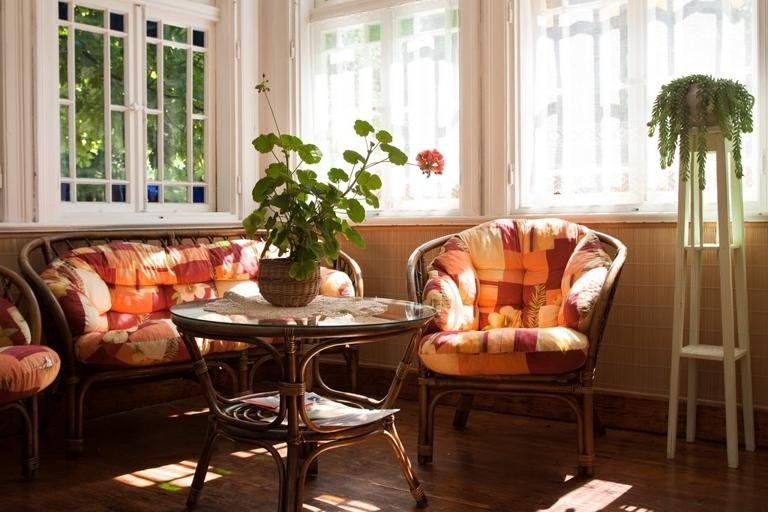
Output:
<box><xmin>664</xmin><ymin>125</ymin><xmax>756</xmax><ymax>470</ymax></box>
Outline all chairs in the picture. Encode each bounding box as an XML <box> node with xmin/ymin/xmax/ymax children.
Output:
<box><xmin>0</xmin><ymin>264</ymin><xmax>63</xmax><ymax>484</ymax></box>
<box><xmin>399</xmin><ymin>212</ymin><xmax>630</xmax><ymax>479</ymax></box>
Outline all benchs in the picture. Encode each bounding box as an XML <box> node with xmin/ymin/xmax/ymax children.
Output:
<box><xmin>17</xmin><ymin>226</ymin><xmax>367</xmax><ymax>456</ymax></box>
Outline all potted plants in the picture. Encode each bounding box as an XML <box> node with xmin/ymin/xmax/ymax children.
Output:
<box><xmin>644</xmin><ymin>74</ymin><xmax>756</xmax><ymax>192</ymax></box>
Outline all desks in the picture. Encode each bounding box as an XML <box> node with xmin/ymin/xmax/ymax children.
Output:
<box><xmin>167</xmin><ymin>295</ymin><xmax>439</xmax><ymax>511</ymax></box>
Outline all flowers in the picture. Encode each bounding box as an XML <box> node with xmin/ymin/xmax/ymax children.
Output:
<box><xmin>240</xmin><ymin>71</ymin><xmax>447</xmax><ymax>282</ymax></box>
